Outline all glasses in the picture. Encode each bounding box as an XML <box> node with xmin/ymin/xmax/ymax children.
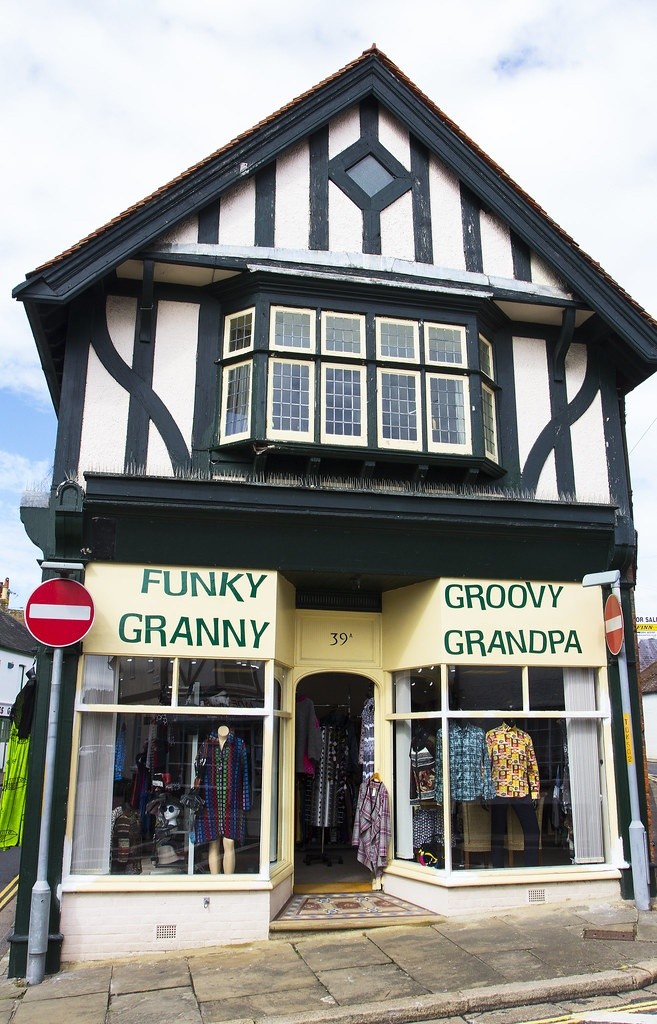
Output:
<box><xmin>162</xmin><ymin>806</ymin><xmax>181</xmax><ymax>813</ymax></box>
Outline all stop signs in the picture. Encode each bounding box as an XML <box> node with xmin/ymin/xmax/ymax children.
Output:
<box><xmin>603</xmin><ymin>594</ymin><xmax>624</xmax><ymax>656</ymax></box>
<box><xmin>24</xmin><ymin>576</ymin><xmax>95</xmax><ymax>647</ymax></box>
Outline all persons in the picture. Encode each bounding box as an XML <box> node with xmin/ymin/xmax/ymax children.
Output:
<box><xmin>411</xmin><ymin>719</ymin><xmax>495</xmax><ymax>806</ymax></box>
<box><xmin>194</xmin><ymin>726</ymin><xmax>251</xmax><ymax>874</ymax></box>
<box><xmin>157</xmin><ymin>799</ymin><xmax>183</xmax><ymax>845</ymax></box>
<box><xmin>481</xmin><ymin>705</ymin><xmax>540</xmax><ymax>868</ymax></box>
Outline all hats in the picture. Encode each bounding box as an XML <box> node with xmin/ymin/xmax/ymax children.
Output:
<box><xmin>157</xmin><ymin>846</ymin><xmax>180</xmax><ymax>864</ymax></box>
<box><xmin>164</xmin><ymin>796</ymin><xmax>184</xmax><ymax>816</ymax></box>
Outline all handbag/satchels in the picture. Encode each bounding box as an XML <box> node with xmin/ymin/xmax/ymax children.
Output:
<box><xmin>180</xmin><ymin>785</ymin><xmax>205</xmax><ymax>813</ymax></box>
